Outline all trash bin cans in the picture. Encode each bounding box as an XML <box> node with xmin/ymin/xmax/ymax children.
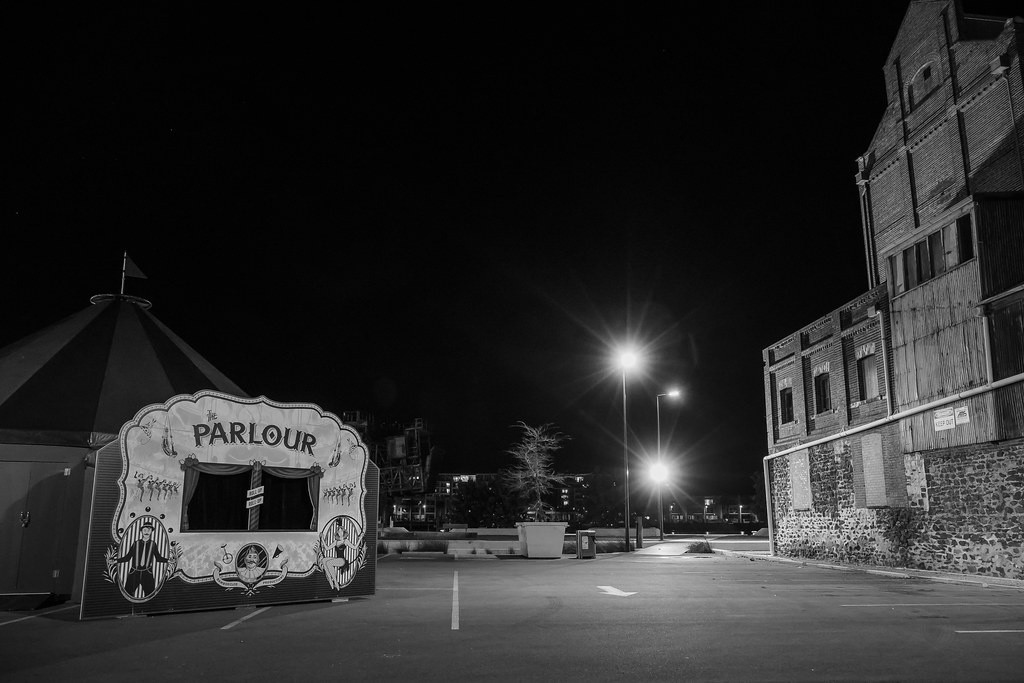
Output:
<box><xmin>576</xmin><ymin>530</ymin><xmax>596</xmax><ymax>559</ymax></box>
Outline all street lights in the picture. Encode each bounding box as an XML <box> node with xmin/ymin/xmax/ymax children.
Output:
<box><xmin>656</xmin><ymin>390</ymin><xmax>679</xmax><ymax>540</ymax></box>
<box><xmin>621</xmin><ymin>349</ymin><xmax>638</xmax><ymax>553</ymax></box>
